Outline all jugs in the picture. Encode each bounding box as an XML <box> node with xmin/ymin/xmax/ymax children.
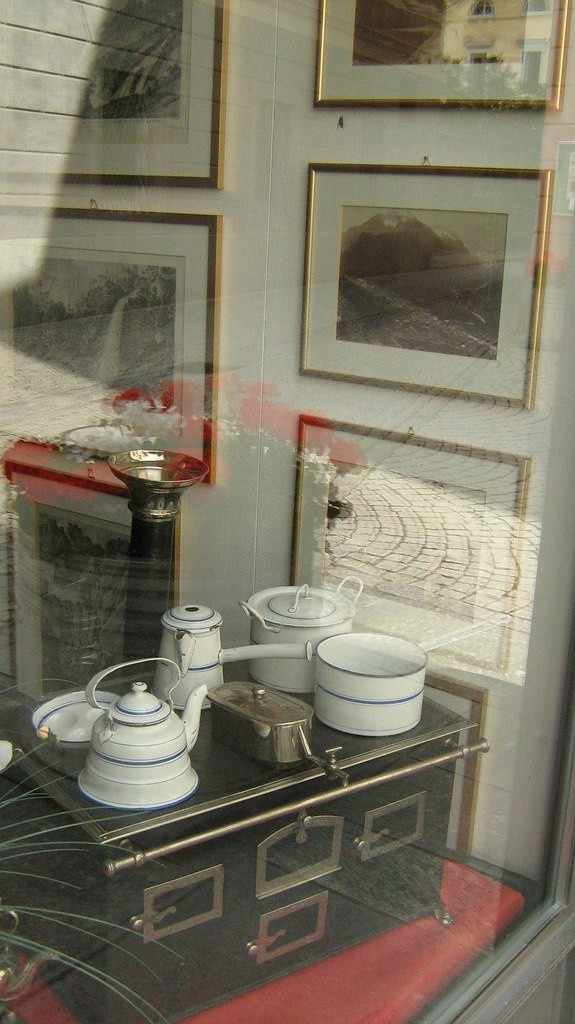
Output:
<box><xmin>149</xmin><ymin>604</ymin><xmax>312</xmax><ymax>711</ymax></box>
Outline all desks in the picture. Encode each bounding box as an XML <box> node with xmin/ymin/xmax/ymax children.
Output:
<box><xmin>0</xmin><ymin>846</ymin><xmax>544</xmax><ymax>1024</ymax></box>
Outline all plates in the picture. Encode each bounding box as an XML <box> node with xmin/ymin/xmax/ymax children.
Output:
<box><xmin>31</xmin><ymin>691</ymin><xmax>123</xmax><ymax>749</ymax></box>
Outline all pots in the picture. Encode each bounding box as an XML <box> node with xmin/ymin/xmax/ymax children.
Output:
<box><xmin>239</xmin><ymin>575</ymin><xmax>363</xmax><ymax>693</ymax></box>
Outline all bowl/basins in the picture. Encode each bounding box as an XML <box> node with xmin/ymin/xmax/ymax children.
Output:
<box><xmin>313</xmin><ymin>633</ymin><xmax>429</xmax><ymax>737</ymax></box>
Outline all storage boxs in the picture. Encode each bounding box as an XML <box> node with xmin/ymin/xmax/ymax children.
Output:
<box><xmin>1</xmin><ymin>682</ymin><xmax>492</xmax><ymax>1024</ymax></box>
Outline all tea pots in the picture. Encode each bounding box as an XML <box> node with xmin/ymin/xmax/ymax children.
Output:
<box><xmin>78</xmin><ymin>658</ymin><xmax>210</xmax><ymax>812</ymax></box>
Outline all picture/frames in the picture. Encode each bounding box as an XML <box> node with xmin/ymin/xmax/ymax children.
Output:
<box><xmin>298</xmin><ymin>163</ymin><xmax>556</xmax><ymax>410</ymax></box>
<box><xmin>289</xmin><ymin>413</ymin><xmax>533</xmax><ymax>675</ymax></box>
<box><xmin>313</xmin><ymin>0</ymin><xmax>571</xmax><ymax>112</ymax></box>
<box><xmin>421</xmin><ymin>669</ymin><xmax>489</xmax><ymax>856</ymax></box>
<box><xmin>3</xmin><ymin>459</ymin><xmax>182</xmax><ymax>703</ymax></box>
<box><xmin>0</xmin><ymin>0</ymin><xmax>230</xmax><ymax>190</ymax></box>
<box><xmin>0</xmin><ymin>205</ymin><xmax>223</xmax><ymax>487</ymax></box>
